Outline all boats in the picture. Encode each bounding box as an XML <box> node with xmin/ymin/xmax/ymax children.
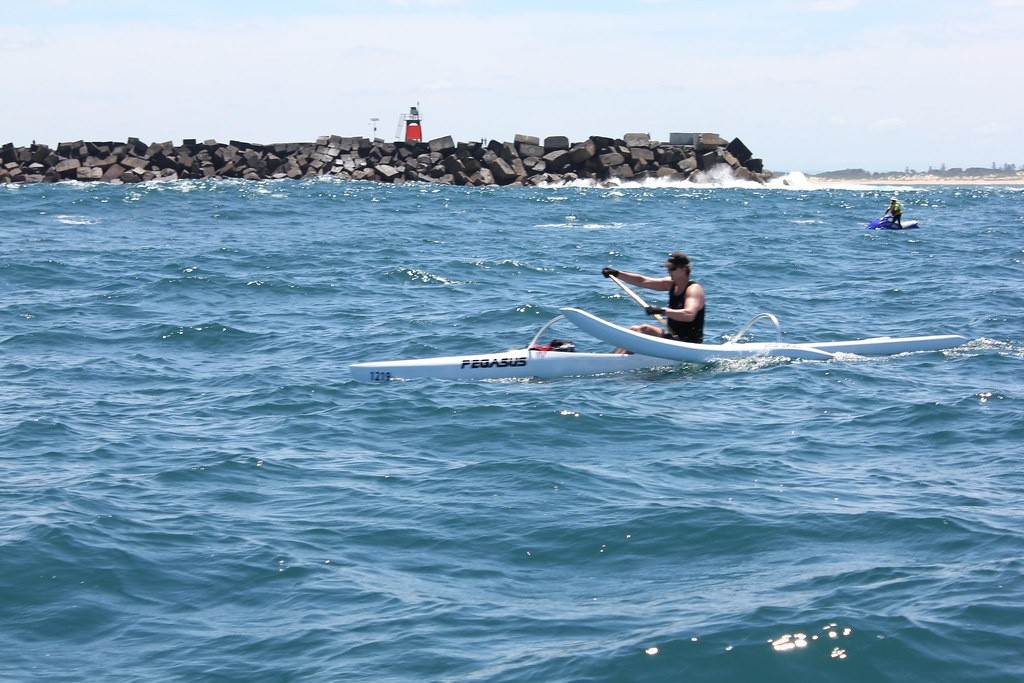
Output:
<box><xmin>559</xmin><ymin>304</ymin><xmax>969</xmax><ymax>364</ymax></box>
<box><xmin>867</xmin><ymin>214</ymin><xmax>917</xmax><ymax>230</ymax></box>
<box><xmin>348</xmin><ymin>315</ymin><xmax>695</xmax><ymax>382</ymax></box>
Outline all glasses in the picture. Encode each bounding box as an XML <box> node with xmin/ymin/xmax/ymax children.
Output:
<box><xmin>667</xmin><ymin>267</ymin><xmax>680</xmax><ymax>271</ymax></box>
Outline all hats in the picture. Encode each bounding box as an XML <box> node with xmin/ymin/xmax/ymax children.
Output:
<box><xmin>890</xmin><ymin>197</ymin><xmax>897</xmax><ymax>201</ymax></box>
<box><xmin>659</xmin><ymin>252</ymin><xmax>690</xmax><ymax>269</ymax></box>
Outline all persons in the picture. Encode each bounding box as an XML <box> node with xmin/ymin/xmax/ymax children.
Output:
<box><xmin>883</xmin><ymin>196</ymin><xmax>903</xmax><ymax>228</ymax></box>
<box><xmin>614</xmin><ymin>252</ymin><xmax>706</xmax><ymax>354</ymax></box>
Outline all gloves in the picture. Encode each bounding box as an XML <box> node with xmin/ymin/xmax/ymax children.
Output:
<box><xmin>602</xmin><ymin>267</ymin><xmax>618</xmax><ymax>279</ymax></box>
<box><xmin>644</xmin><ymin>305</ymin><xmax>666</xmax><ymax>316</ymax></box>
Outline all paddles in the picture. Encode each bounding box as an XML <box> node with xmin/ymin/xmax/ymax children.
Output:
<box><xmin>604</xmin><ymin>269</ymin><xmax>680</xmax><ymax>340</ymax></box>
